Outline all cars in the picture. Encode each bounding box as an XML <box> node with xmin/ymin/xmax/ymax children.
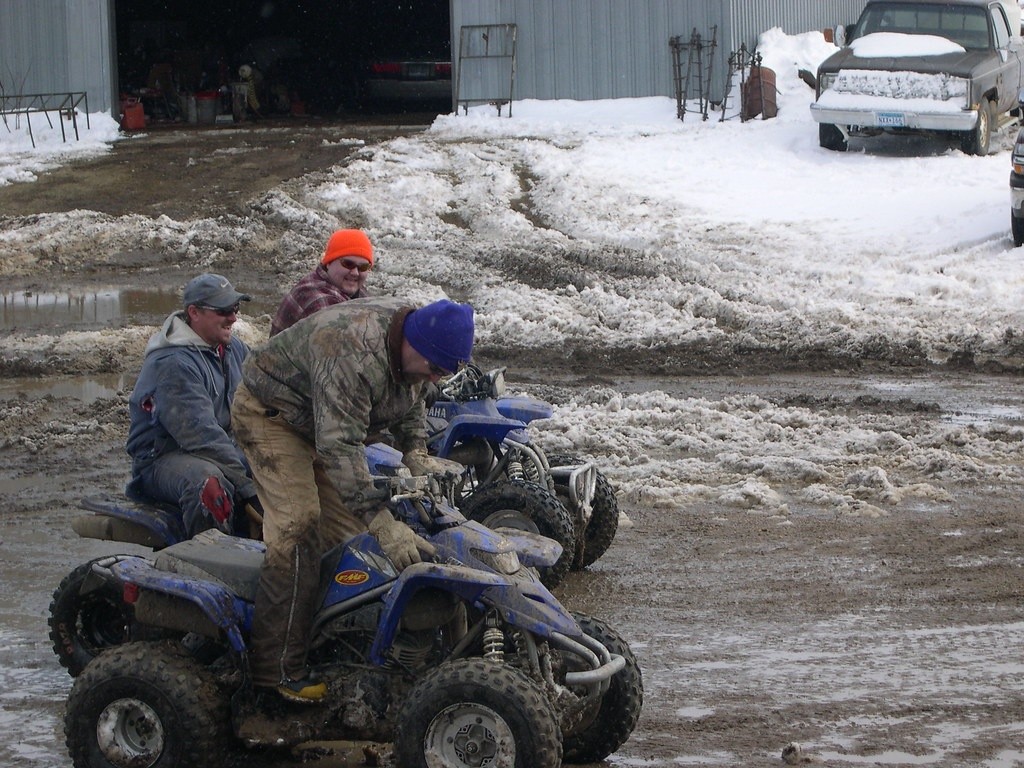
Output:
<box><xmin>362</xmin><ymin>52</ymin><xmax>454</xmax><ymax>106</ymax></box>
<box><xmin>810</xmin><ymin>0</ymin><xmax>1024</xmax><ymax>158</ymax></box>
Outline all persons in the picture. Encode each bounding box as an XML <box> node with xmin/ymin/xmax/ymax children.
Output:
<box><xmin>230</xmin><ymin>294</ymin><xmax>478</xmax><ymax>704</ymax></box>
<box><xmin>123</xmin><ymin>273</ymin><xmax>262</xmax><ymax>537</ymax></box>
<box><xmin>267</xmin><ymin>230</ymin><xmax>441</xmax><ymax>410</ymax></box>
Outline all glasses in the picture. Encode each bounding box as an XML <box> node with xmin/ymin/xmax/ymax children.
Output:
<box><xmin>428</xmin><ymin>361</ymin><xmax>450</xmax><ymax>377</ymax></box>
<box><xmin>198</xmin><ymin>303</ymin><xmax>240</xmax><ymax>316</ymax></box>
<box><xmin>339</xmin><ymin>257</ymin><xmax>370</xmax><ymax>272</ymax></box>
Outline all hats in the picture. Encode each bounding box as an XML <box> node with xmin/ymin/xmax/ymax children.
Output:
<box><xmin>403</xmin><ymin>299</ymin><xmax>474</xmax><ymax>375</ymax></box>
<box><xmin>322</xmin><ymin>229</ymin><xmax>373</xmax><ymax>269</ymax></box>
<box><xmin>183</xmin><ymin>274</ymin><xmax>252</xmax><ymax>308</ymax></box>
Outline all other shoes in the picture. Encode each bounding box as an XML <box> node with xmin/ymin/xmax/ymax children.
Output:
<box><xmin>255</xmin><ymin>680</ymin><xmax>328</xmax><ymax>705</ymax></box>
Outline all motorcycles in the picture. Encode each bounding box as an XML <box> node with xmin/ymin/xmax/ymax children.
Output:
<box><xmin>42</xmin><ymin>441</ymin><xmax>652</xmax><ymax>768</ymax></box>
<box><xmin>75</xmin><ymin>364</ymin><xmax>618</xmax><ymax>594</ymax></box>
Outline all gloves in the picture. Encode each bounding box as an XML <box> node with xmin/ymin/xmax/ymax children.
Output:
<box><xmin>241</xmin><ymin>495</ymin><xmax>263</xmax><ymax>525</ymax></box>
<box><xmin>402</xmin><ymin>448</ymin><xmax>467</xmax><ymax>504</ymax></box>
<box><xmin>367</xmin><ymin>508</ymin><xmax>437</xmax><ymax>570</ymax></box>
